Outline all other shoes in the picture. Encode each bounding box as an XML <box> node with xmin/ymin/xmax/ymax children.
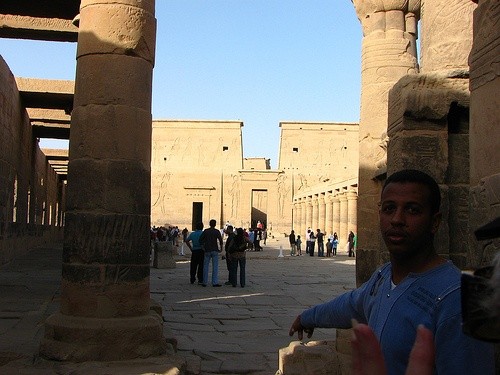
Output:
<box><xmin>232</xmin><ymin>284</ymin><xmax>245</xmax><ymax>288</ymax></box>
<box><xmin>290</xmin><ymin>254</ymin><xmax>296</xmax><ymax>257</ymax></box>
<box><xmin>190</xmin><ymin>281</ymin><xmax>202</xmax><ymax>285</ymax></box>
<box><xmin>202</xmin><ymin>283</ymin><xmax>222</xmax><ymax>287</ymax></box>
<box><xmin>246</xmin><ymin>248</ymin><xmax>264</xmax><ymax>252</ymax></box>
<box><xmin>297</xmin><ymin>254</ymin><xmax>302</xmax><ymax>256</ymax></box>
<box><xmin>307</xmin><ymin>252</ymin><xmax>310</xmax><ymax>254</ymax></box>
<box><xmin>310</xmin><ymin>255</ymin><xmax>337</xmax><ymax>258</ymax></box>
<box><xmin>225</xmin><ymin>282</ymin><xmax>232</xmax><ymax>285</ymax></box>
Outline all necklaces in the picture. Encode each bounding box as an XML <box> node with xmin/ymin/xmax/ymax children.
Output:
<box><xmin>225</xmin><ymin>226</ymin><xmax>238</xmax><ymax>285</ymax></box>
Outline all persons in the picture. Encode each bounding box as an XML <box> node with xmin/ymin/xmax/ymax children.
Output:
<box><xmin>227</xmin><ymin>228</ymin><xmax>252</xmax><ymax>287</ymax></box>
<box><xmin>326</xmin><ymin>239</ymin><xmax>333</xmax><ymax>257</ymax></box>
<box><xmin>150</xmin><ymin>225</ymin><xmax>180</xmax><ymax>255</ymax></box>
<box><xmin>289</xmin><ymin>230</ymin><xmax>296</xmax><ymax>257</ymax></box>
<box><xmin>347</xmin><ymin>231</ymin><xmax>357</xmax><ymax>257</ymax></box>
<box><xmin>289</xmin><ymin>169</ymin><xmax>492</xmax><ymax>375</ymax></box>
<box><xmin>332</xmin><ymin>236</ymin><xmax>339</xmax><ymax>257</ymax></box>
<box><xmin>182</xmin><ymin>228</ymin><xmax>188</xmax><ymax>242</ymax></box>
<box><xmin>186</xmin><ymin>222</ymin><xmax>209</xmax><ymax>284</ymax></box>
<box><xmin>332</xmin><ymin>232</ymin><xmax>338</xmax><ymax>241</ymax></box>
<box><xmin>316</xmin><ymin>229</ymin><xmax>326</xmax><ymax>257</ymax></box>
<box><xmin>310</xmin><ymin>232</ymin><xmax>317</xmax><ymax>256</ymax></box>
<box><xmin>306</xmin><ymin>227</ymin><xmax>312</xmax><ymax>254</ymax></box>
<box><xmin>329</xmin><ymin>233</ymin><xmax>334</xmax><ymax>243</ymax></box>
<box><xmin>296</xmin><ymin>235</ymin><xmax>302</xmax><ymax>256</ymax></box>
<box><xmin>222</xmin><ymin>219</ymin><xmax>268</xmax><ymax>251</ymax></box>
<box><xmin>199</xmin><ymin>219</ymin><xmax>223</xmax><ymax>287</ymax></box>
